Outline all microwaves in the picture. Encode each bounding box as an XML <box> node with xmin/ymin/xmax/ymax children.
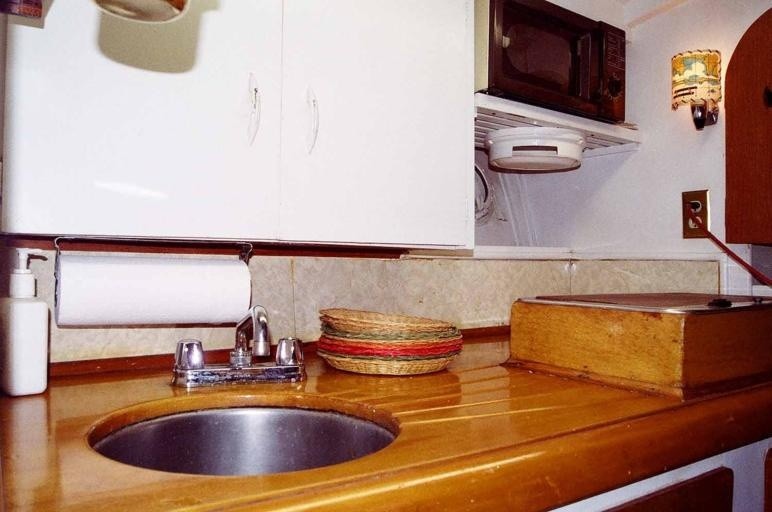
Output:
<box><xmin>474</xmin><ymin>0</ymin><xmax>626</xmax><ymax>126</ymax></box>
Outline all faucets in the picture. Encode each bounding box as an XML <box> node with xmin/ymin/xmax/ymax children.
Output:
<box><xmin>227</xmin><ymin>303</ymin><xmax>273</xmax><ymax>364</ymax></box>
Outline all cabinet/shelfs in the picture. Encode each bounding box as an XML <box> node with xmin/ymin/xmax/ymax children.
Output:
<box><xmin>2</xmin><ymin>0</ymin><xmax>472</xmax><ymax>251</ymax></box>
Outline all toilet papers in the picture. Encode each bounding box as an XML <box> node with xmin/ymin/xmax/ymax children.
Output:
<box><xmin>54</xmin><ymin>253</ymin><xmax>252</xmax><ymax>327</ymax></box>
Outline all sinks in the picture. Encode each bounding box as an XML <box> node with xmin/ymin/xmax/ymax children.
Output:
<box><xmin>84</xmin><ymin>393</ymin><xmax>402</xmax><ymax>474</ymax></box>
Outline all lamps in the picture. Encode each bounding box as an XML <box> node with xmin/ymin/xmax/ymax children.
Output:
<box><xmin>670</xmin><ymin>49</ymin><xmax>721</xmax><ymax>132</ymax></box>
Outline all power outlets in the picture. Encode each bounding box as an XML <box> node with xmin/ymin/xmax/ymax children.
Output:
<box><xmin>681</xmin><ymin>189</ymin><xmax>710</xmax><ymax>239</ymax></box>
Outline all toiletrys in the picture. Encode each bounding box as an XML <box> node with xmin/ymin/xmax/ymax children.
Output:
<box><xmin>1</xmin><ymin>247</ymin><xmax>50</xmax><ymax>398</ymax></box>
